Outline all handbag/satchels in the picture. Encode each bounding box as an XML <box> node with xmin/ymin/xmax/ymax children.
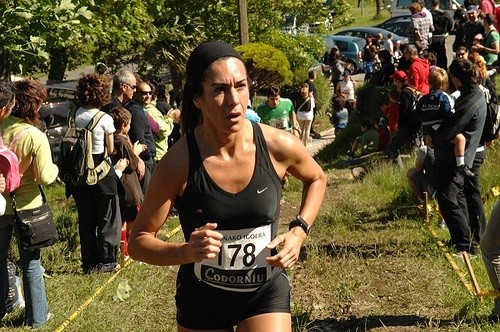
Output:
<box><xmin>15</xmin><ymin>203</ymin><xmax>57</xmax><ymax>250</ymax></box>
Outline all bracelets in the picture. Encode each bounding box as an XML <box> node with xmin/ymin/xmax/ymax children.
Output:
<box><xmin>289</xmin><ymin>215</ymin><xmax>310</xmax><ymax>236</ymax></box>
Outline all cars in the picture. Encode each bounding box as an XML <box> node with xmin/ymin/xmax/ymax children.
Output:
<box><xmin>329</xmin><ymin>36</ymin><xmax>366</xmax><ymax>75</ymax></box>
<box><xmin>375</xmin><ymin>16</ymin><xmax>412</xmax><ymax>37</ymax></box>
<box><xmin>333</xmin><ymin>27</ymin><xmax>410</xmax><ymax>51</ymax></box>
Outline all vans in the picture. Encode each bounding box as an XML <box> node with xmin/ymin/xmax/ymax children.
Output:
<box><xmin>390</xmin><ymin>0</ymin><xmax>464</xmax><ymax>35</ymax></box>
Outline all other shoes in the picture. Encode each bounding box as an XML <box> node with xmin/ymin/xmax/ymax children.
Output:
<box><xmin>455</xmin><ymin>165</ymin><xmax>475</xmax><ymax>177</ymax></box>
<box><xmin>347</xmin><ymin>152</ymin><xmax>352</xmax><ymax>157</ymax></box>
<box><xmin>446</xmin><ymin>240</ymin><xmax>479</xmax><ymax>255</ymax></box>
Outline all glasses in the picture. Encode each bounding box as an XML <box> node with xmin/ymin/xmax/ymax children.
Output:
<box><xmin>121</xmin><ymin>83</ymin><xmax>137</xmax><ymax>90</ymax></box>
<box><xmin>457</xmin><ymin>53</ymin><xmax>466</xmax><ymax>56</ymax></box>
<box><xmin>137</xmin><ymin>90</ymin><xmax>153</xmax><ymax>95</ymax></box>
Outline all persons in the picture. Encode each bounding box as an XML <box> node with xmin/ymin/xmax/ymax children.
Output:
<box><xmin>328</xmin><ymin>0</ymin><xmax>500</xmax><ymax>159</ymax></box>
<box><xmin>256</xmin><ymin>85</ymin><xmax>299</xmax><ymax>137</ymax></box>
<box><xmin>417</xmin><ymin>68</ymin><xmax>474</xmax><ymax>177</ymax></box>
<box><xmin>480</xmin><ymin>198</ymin><xmax>500</xmax><ymax>315</ymax></box>
<box><xmin>295</xmin><ymin>83</ymin><xmax>315</xmax><ymax>147</ymax></box>
<box><xmin>126</xmin><ymin>41</ymin><xmax>326</xmax><ymax>332</ymax></box>
<box><xmin>66</xmin><ymin>68</ymin><xmax>181</xmax><ymax>273</ymax></box>
<box><xmin>0</xmin><ymin>78</ymin><xmax>59</xmax><ymax>328</ymax></box>
<box><xmin>426</xmin><ymin>51</ymin><xmax>487</xmax><ymax>254</ymax></box>
<box><xmin>305</xmin><ymin>71</ymin><xmax>318</xmax><ymax>134</ymax></box>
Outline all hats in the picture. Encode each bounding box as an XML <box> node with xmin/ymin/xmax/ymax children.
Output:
<box><xmin>466</xmin><ymin>5</ymin><xmax>477</xmax><ymax>14</ymax></box>
<box><xmin>389</xmin><ymin>71</ymin><xmax>408</xmax><ymax>80</ymax></box>
<box><xmin>374</xmin><ymin>115</ymin><xmax>386</xmax><ymax>126</ymax></box>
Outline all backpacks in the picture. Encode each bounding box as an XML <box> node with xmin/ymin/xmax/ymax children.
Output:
<box><xmin>480</xmin><ymin>98</ymin><xmax>500</xmax><ymax>144</ymax></box>
<box><xmin>57</xmin><ymin>105</ymin><xmax>111</xmax><ymax>186</ymax></box>
